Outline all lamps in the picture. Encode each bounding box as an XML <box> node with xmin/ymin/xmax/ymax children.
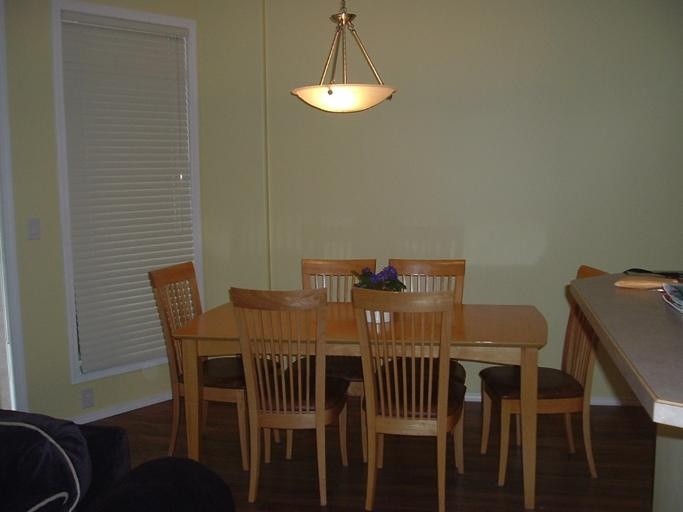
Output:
<box><xmin>289</xmin><ymin>0</ymin><xmax>396</xmax><ymax>114</ymax></box>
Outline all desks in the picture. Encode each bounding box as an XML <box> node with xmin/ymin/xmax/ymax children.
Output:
<box><xmin>564</xmin><ymin>271</ymin><xmax>682</xmax><ymax>512</ymax></box>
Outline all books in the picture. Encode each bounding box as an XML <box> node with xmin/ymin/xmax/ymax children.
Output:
<box><xmin>660</xmin><ymin>291</ymin><xmax>682</xmax><ymax>313</ymax></box>
<box><xmin>662</xmin><ymin>282</ymin><xmax>682</xmax><ymax>306</ymax></box>
